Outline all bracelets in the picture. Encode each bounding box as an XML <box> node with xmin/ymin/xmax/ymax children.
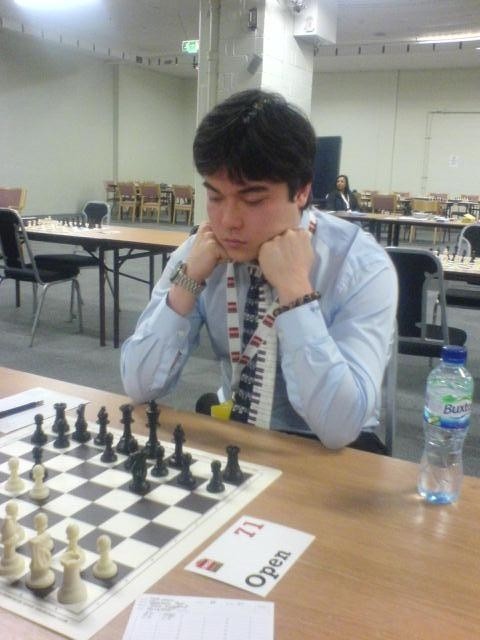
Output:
<box><xmin>273</xmin><ymin>291</ymin><xmax>321</xmax><ymax>318</ymax></box>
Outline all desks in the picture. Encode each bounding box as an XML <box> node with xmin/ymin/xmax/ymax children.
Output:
<box><xmin>388</xmin><ymin>245</ymin><xmax>480</xmax><ymax>288</ymax></box>
<box><xmin>15</xmin><ymin>220</ymin><xmax>193</xmax><ymax>348</ymax></box>
<box><xmin>0</xmin><ymin>366</ymin><xmax>480</xmax><ymax>640</ymax></box>
<box><xmin>337</xmin><ymin>210</ymin><xmax>480</xmax><ymax>247</ymax></box>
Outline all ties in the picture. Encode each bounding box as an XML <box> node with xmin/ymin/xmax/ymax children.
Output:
<box><xmin>230</xmin><ymin>262</ymin><xmax>268</xmax><ymax>431</ymax></box>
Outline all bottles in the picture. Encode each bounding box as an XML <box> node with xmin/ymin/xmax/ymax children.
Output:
<box><xmin>417</xmin><ymin>345</ymin><xmax>475</xmax><ymax>505</ymax></box>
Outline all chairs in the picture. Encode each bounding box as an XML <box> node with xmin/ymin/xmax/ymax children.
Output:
<box><xmin>0</xmin><ymin>208</ymin><xmax>81</xmax><ymax>347</ymax></box>
<box><xmin>430</xmin><ymin>192</ymin><xmax>447</xmax><ymax>202</ymax></box>
<box><xmin>118</xmin><ymin>182</ymin><xmax>140</xmax><ymax>223</ymax></box>
<box><xmin>172</xmin><ymin>184</ymin><xmax>195</xmax><ymax>226</ymax></box>
<box><xmin>410</xmin><ymin>199</ymin><xmax>442</xmax><ymax>245</ymax></box>
<box><xmin>374</xmin><ymin>321</ymin><xmax>400</xmax><ymax>456</ymax></box>
<box><xmin>102</xmin><ymin>180</ymin><xmax>118</xmax><ymax>213</ymax></box>
<box><xmin>141</xmin><ymin>183</ymin><xmax>168</xmax><ymax>224</ymax></box>
<box><xmin>365</xmin><ymin>190</ymin><xmax>378</xmax><ymax>197</ymax></box>
<box><xmin>371</xmin><ymin>195</ymin><xmax>397</xmax><ymax>213</ymax></box>
<box><xmin>384</xmin><ymin>246</ymin><xmax>467</xmax><ymax>371</ymax></box>
<box><xmin>35</xmin><ymin>200</ymin><xmax>124</xmax><ymax>318</ymax></box>
<box><xmin>462</xmin><ymin>194</ymin><xmax>479</xmax><ymax>202</ymax></box>
<box><xmin>0</xmin><ymin>186</ymin><xmax>27</xmax><ymax>212</ymax></box>
<box><xmin>393</xmin><ymin>191</ymin><xmax>410</xmax><ymax>198</ymax></box>
<box><xmin>428</xmin><ymin>224</ymin><xmax>480</xmax><ymax>372</ymax></box>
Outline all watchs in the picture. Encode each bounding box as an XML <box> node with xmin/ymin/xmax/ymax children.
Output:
<box><xmin>169</xmin><ymin>259</ymin><xmax>185</xmax><ymax>282</ymax></box>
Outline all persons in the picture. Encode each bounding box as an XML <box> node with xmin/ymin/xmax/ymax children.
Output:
<box><xmin>326</xmin><ymin>175</ymin><xmax>363</xmax><ymax>229</ymax></box>
<box><xmin>120</xmin><ymin>88</ymin><xmax>399</xmax><ymax>451</ymax></box>
